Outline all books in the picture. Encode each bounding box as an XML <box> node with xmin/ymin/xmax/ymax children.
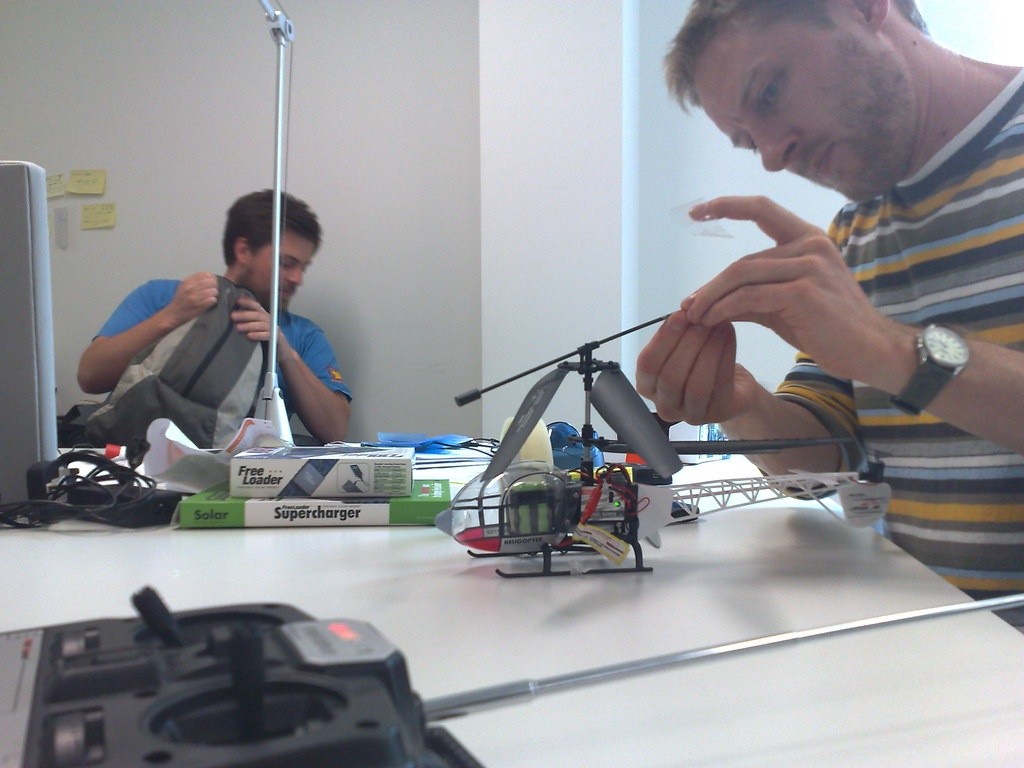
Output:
<box><xmin>179</xmin><ymin>446</ymin><xmax>452</xmax><ymax>529</ymax></box>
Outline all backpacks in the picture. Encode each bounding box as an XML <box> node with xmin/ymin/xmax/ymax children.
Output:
<box><xmin>75</xmin><ymin>275</ymin><xmax>269</xmax><ymax>467</ymax></box>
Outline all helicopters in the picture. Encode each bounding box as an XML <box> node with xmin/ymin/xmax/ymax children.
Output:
<box><xmin>431</xmin><ymin>299</ymin><xmax>882</xmax><ymax>580</ymax></box>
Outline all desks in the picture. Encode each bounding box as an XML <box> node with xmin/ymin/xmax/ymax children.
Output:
<box><xmin>1</xmin><ymin>443</ymin><xmax>1024</xmax><ymax>768</ymax></box>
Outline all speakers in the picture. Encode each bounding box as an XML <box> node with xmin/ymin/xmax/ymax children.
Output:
<box><xmin>0</xmin><ymin>160</ymin><xmax>66</xmax><ymax>504</ymax></box>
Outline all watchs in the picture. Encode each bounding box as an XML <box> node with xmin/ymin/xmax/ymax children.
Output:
<box><xmin>891</xmin><ymin>323</ymin><xmax>969</xmax><ymax>416</ymax></box>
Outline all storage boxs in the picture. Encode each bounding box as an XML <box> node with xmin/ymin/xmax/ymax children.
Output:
<box><xmin>179</xmin><ymin>480</ymin><xmax>451</xmax><ymax>529</ymax></box>
<box><xmin>669</xmin><ymin>421</ymin><xmax>729</xmax><ymax>464</ymax></box>
<box><xmin>230</xmin><ymin>447</ymin><xmax>416</xmax><ymax>498</ymax></box>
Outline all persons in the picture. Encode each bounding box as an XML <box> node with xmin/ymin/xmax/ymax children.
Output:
<box><xmin>635</xmin><ymin>0</ymin><xmax>1024</xmax><ymax>629</ymax></box>
<box><xmin>74</xmin><ymin>190</ymin><xmax>353</xmax><ymax>444</ymax></box>
